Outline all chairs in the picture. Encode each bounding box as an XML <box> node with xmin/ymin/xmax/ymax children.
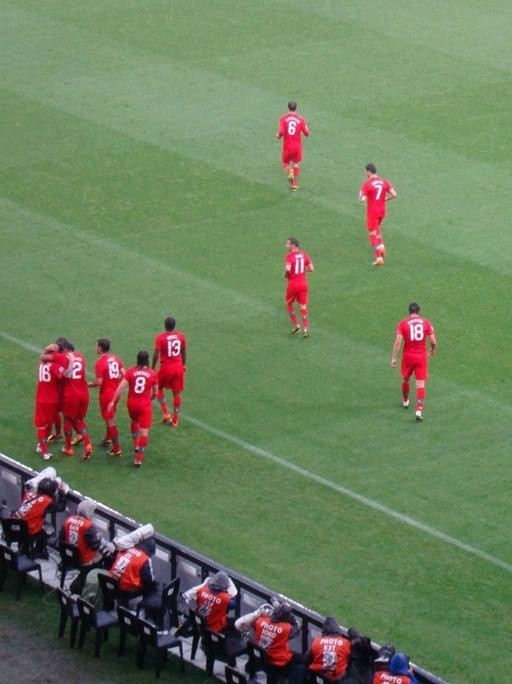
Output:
<box><xmin>1</xmin><ymin>511</ymin><xmax>343</xmax><ymax>684</ymax></box>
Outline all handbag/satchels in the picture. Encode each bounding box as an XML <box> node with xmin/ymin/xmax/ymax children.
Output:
<box><xmin>82</xmin><ymin>568</ymin><xmax>110</xmax><ymax>611</ymax></box>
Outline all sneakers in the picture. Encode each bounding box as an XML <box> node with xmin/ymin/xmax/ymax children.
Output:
<box><xmin>134</xmin><ymin>458</ymin><xmax>142</xmax><ymax>464</ymax></box>
<box><xmin>415</xmin><ymin>414</ymin><xmax>423</xmax><ymax>422</ymax></box>
<box><xmin>292</xmin><ymin>326</ymin><xmax>300</xmax><ymax>335</ymax></box>
<box><xmin>402</xmin><ymin>399</ymin><xmax>410</xmax><ymax>406</ymax></box>
<box><xmin>303</xmin><ymin>330</ymin><xmax>311</xmax><ymax>337</ymax></box>
<box><xmin>37</xmin><ymin>433</ymin><xmax>122</xmax><ymax>460</ymax></box>
<box><xmin>163</xmin><ymin>414</ymin><xmax>178</xmax><ymax>427</ymax></box>
<box><xmin>372</xmin><ymin>249</ymin><xmax>384</xmax><ymax>265</ymax></box>
<box><xmin>287</xmin><ymin>169</ymin><xmax>299</xmax><ymax>190</ymax></box>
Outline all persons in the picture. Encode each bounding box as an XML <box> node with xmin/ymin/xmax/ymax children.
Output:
<box><xmin>62</xmin><ymin>500</ymin><xmax>160</xmax><ymax>611</ymax></box>
<box><xmin>33</xmin><ymin>316</ymin><xmax>187</xmax><ymax>466</ymax></box>
<box><xmin>15</xmin><ymin>466</ymin><xmax>70</xmax><ymax>559</ymax></box>
<box><xmin>358</xmin><ymin>163</ymin><xmax>397</xmax><ymax>267</ymax></box>
<box><xmin>282</xmin><ymin>235</ymin><xmax>315</xmax><ymax>340</ymax></box>
<box><xmin>234</xmin><ymin>601</ymin><xmax>418</xmax><ymax>683</ymax></box>
<box><xmin>276</xmin><ymin>100</ymin><xmax>311</xmax><ymax>193</ymax></box>
<box><xmin>391</xmin><ymin>303</ymin><xmax>436</xmax><ymax>422</ymax></box>
<box><xmin>183</xmin><ymin>570</ymin><xmax>241</xmax><ymax>646</ymax></box>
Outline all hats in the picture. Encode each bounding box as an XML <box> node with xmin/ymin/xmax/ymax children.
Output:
<box><xmin>78</xmin><ymin>501</ymin><xmax>96</xmax><ymax>517</ymax></box>
<box><xmin>209</xmin><ymin>570</ymin><xmax>228</xmax><ymax>590</ymax></box>
<box><xmin>38</xmin><ymin>478</ymin><xmax>58</xmax><ymax>495</ymax></box>
